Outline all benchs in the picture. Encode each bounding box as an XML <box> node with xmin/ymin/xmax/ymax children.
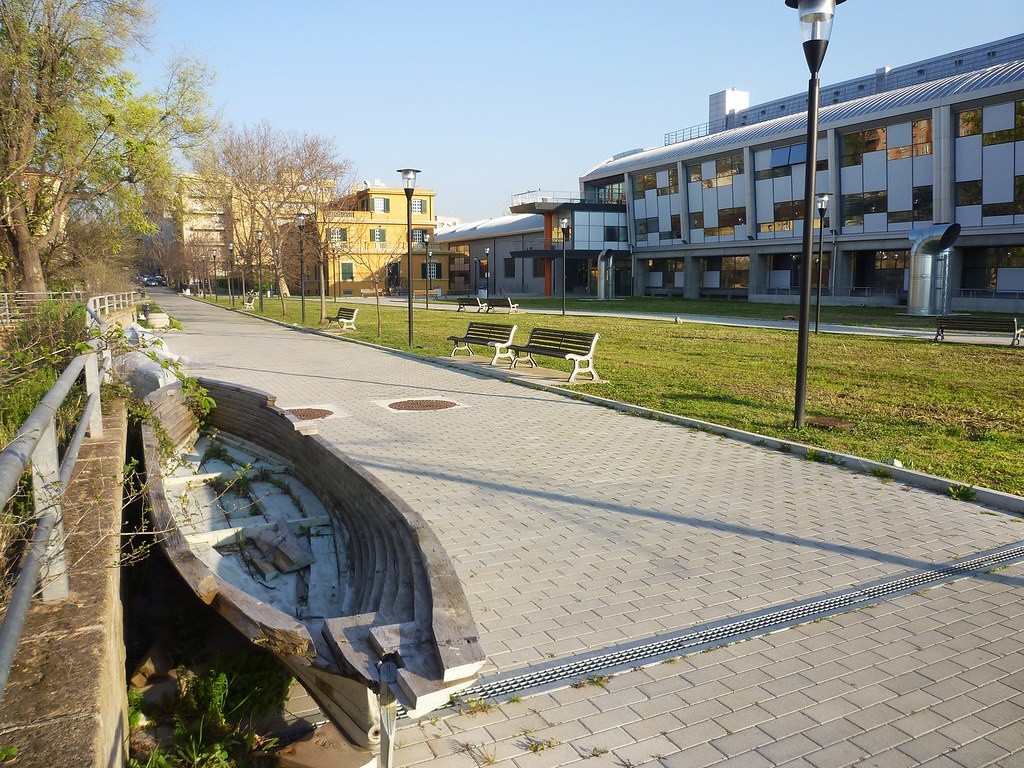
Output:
<box><xmin>196</xmin><ymin>291</ymin><xmax>203</xmax><ymax>298</ymax></box>
<box><xmin>485</xmin><ymin>297</ymin><xmax>519</xmax><ymax>314</ymax></box>
<box><xmin>241</xmin><ymin>297</ymin><xmax>255</xmax><ymax>310</ymax></box>
<box><xmin>414</xmin><ymin>290</ymin><xmax>438</xmax><ymax>298</ymax></box>
<box><xmin>361</xmin><ymin>288</ymin><xmax>385</xmax><ymax>297</ymax></box>
<box><xmin>325</xmin><ymin>307</ymin><xmax>359</xmax><ymax>331</ymax></box>
<box><xmin>456</xmin><ymin>297</ymin><xmax>485</xmax><ymax>312</ymax></box>
<box><xmin>447</xmin><ymin>320</ymin><xmax>518</xmax><ymax>366</ymax></box>
<box><xmin>445</xmin><ymin>289</ymin><xmax>472</xmax><ymax>299</ymax></box>
<box><xmin>934</xmin><ymin>314</ymin><xmax>1023</xmax><ymax>345</ymax></box>
<box><xmin>507</xmin><ymin>326</ymin><xmax>602</xmax><ymax>381</ymax></box>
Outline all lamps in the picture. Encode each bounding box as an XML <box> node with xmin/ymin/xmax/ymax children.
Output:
<box><xmin>747</xmin><ymin>235</ymin><xmax>755</xmax><ymax>242</ymax></box>
<box><xmin>682</xmin><ymin>240</ymin><xmax>689</xmax><ymax>245</ymax></box>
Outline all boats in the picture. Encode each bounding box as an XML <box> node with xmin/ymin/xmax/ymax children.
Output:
<box><xmin>139</xmin><ymin>374</ymin><xmax>485</xmax><ymax>752</ymax></box>
<box><xmin>114</xmin><ymin>350</ymin><xmax>175</xmax><ymax>403</ymax></box>
<box><xmin>124</xmin><ymin>323</ymin><xmax>177</xmax><ymax>365</ymax></box>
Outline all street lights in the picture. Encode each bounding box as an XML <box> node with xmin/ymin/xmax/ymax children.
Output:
<box><xmin>253</xmin><ymin>230</ymin><xmax>265</xmax><ymax>313</ymax></box>
<box><xmin>427</xmin><ymin>251</ymin><xmax>433</xmax><ymax>290</ymax></box>
<box><xmin>397</xmin><ymin>169</ymin><xmax>422</xmax><ymax>348</ymax></box>
<box><xmin>331</xmin><ymin>242</ymin><xmax>337</xmax><ymax>303</ymax></box>
<box><xmin>483</xmin><ymin>246</ymin><xmax>490</xmax><ymax>298</ymax></box>
<box><xmin>227</xmin><ymin>242</ymin><xmax>234</xmax><ymax>307</ymax></box>
<box><xmin>200</xmin><ymin>254</ymin><xmax>206</xmax><ymax>298</ymax></box>
<box><xmin>474</xmin><ymin>257</ymin><xmax>477</xmax><ymax>296</ymax></box>
<box><xmin>814</xmin><ymin>191</ymin><xmax>835</xmax><ymax>335</ymax></box>
<box><xmin>423</xmin><ymin>233</ymin><xmax>431</xmax><ymax>309</ymax></box>
<box><xmin>558</xmin><ymin>218</ymin><xmax>568</xmax><ymax>316</ymax></box>
<box><xmin>295</xmin><ymin>212</ymin><xmax>306</xmax><ymax>324</ymax></box>
<box><xmin>785</xmin><ymin>0</ymin><xmax>848</xmax><ymax>430</ymax></box>
<box><xmin>210</xmin><ymin>249</ymin><xmax>218</xmax><ymax>301</ymax></box>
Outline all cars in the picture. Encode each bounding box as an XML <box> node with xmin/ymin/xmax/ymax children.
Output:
<box><xmin>133</xmin><ymin>274</ymin><xmax>167</xmax><ymax>287</ymax></box>
